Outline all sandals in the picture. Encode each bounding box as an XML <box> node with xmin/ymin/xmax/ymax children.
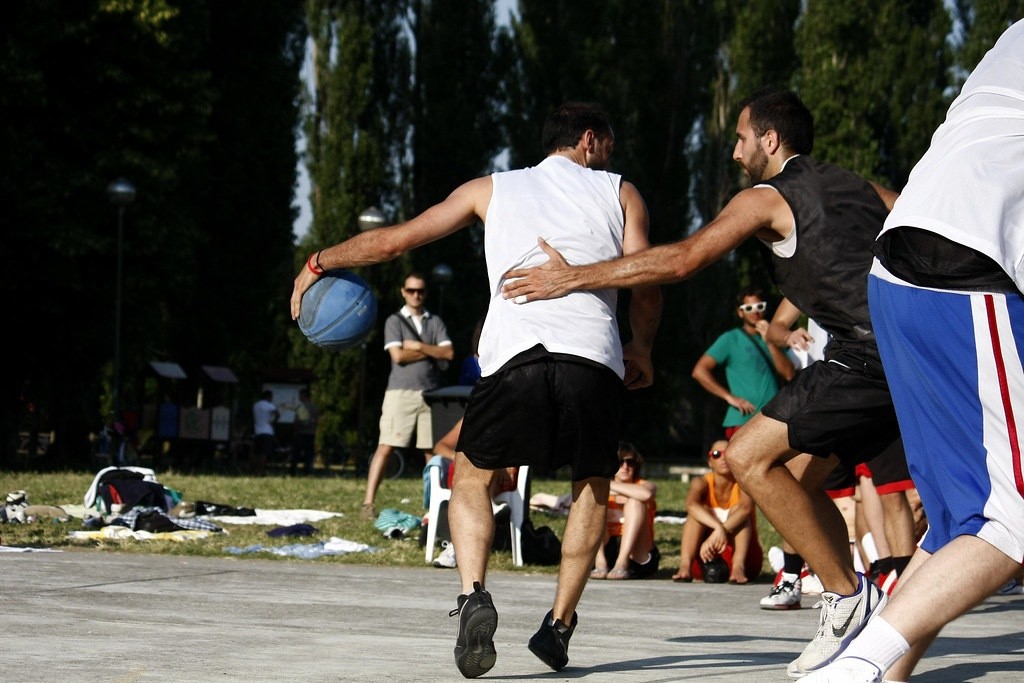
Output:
<box><xmin>588</xmin><ymin>569</ymin><xmax>607</xmax><ymax>579</ymax></box>
<box><xmin>729</xmin><ymin>571</ymin><xmax>747</xmax><ymax>584</ymax></box>
<box><xmin>673</xmin><ymin>567</ymin><xmax>693</xmax><ymax>583</ymax></box>
<box><xmin>606</xmin><ymin>569</ymin><xmax>630</xmax><ymax>579</ymax></box>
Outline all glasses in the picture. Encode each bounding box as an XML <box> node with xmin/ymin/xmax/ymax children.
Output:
<box><xmin>404</xmin><ymin>288</ymin><xmax>426</xmax><ymax>295</ymax></box>
<box><xmin>708</xmin><ymin>449</ymin><xmax>726</xmax><ymax>460</ymax></box>
<box><xmin>741</xmin><ymin>302</ymin><xmax>767</xmax><ymax>314</ymax></box>
<box><xmin>619</xmin><ymin>458</ymin><xmax>637</xmax><ymax>468</ymax></box>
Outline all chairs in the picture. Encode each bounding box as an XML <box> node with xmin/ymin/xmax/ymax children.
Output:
<box><xmin>426</xmin><ymin>464</ymin><xmax>532</xmax><ymax>568</ymax></box>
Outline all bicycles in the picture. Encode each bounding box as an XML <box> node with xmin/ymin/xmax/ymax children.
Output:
<box><xmin>367</xmin><ymin>433</ymin><xmax>406</xmax><ymax>481</ymax></box>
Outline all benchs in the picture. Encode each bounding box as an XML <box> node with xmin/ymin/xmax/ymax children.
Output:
<box><xmin>668</xmin><ymin>465</ymin><xmax>710</xmax><ymax>482</ymax></box>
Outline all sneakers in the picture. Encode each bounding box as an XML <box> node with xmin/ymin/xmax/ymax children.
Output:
<box><xmin>433</xmin><ymin>540</ymin><xmax>458</xmax><ymax>568</ymax></box>
<box><xmin>448</xmin><ymin>581</ymin><xmax>499</xmax><ymax>679</ymax></box>
<box><xmin>528</xmin><ymin>608</ymin><xmax>578</xmax><ymax>672</ymax></box>
<box><xmin>787</xmin><ymin>572</ymin><xmax>888</xmax><ymax>678</ymax></box>
<box><xmin>759</xmin><ymin>571</ymin><xmax>802</xmax><ymax>609</ymax></box>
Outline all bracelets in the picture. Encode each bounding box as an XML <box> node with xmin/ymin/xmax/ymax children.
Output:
<box><xmin>317</xmin><ymin>248</ymin><xmax>329</xmax><ymax>271</ymax></box>
<box><xmin>307</xmin><ymin>252</ymin><xmax>321</xmax><ymax>275</ymax></box>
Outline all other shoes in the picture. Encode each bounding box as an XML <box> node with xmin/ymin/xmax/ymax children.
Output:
<box><xmin>802</xmin><ymin>575</ymin><xmax>825</xmax><ymax>595</ymax></box>
<box><xmin>794</xmin><ymin>656</ymin><xmax>882</xmax><ymax>683</ymax></box>
<box><xmin>865</xmin><ymin>568</ymin><xmax>898</xmax><ymax>596</ymax></box>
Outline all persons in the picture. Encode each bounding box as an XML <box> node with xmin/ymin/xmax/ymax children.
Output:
<box><xmin>291</xmin><ymin>107</ymin><xmax>649</xmax><ymax>680</ymax></box>
<box><xmin>798</xmin><ymin>17</ymin><xmax>1024</xmax><ymax>683</ymax></box>
<box><xmin>500</xmin><ymin>91</ymin><xmax>930</xmax><ymax>676</ymax></box>
<box><xmin>586</xmin><ymin>441</ymin><xmax>661</xmax><ymax>578</ymax></box>
<box><xmin>359</xmin><ymin>269</ymin><xmax>454</xmax><ymax>521</ymax></box>
<box><xmin>289</xmin><ymin>389</ymin><xmax>316</xmax><ymax>476</ymax></box>
<box><xmin>672</xmin><ymin>436</ymin><xmax>762</xmax><ymax>586</ymax></box>
<box><xmin>253</xmin><ymin>391</ymin><xmax>279</xmax><ymax>474</ymax></box>
<box><xmin>99</xmin><ymin>406</ymin><xmax>139</xmax><ymax>460</ymax></box>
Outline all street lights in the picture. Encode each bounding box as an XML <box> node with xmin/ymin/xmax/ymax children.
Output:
<box><xmin>356</xmin><ymin>206</ymin><xmax>385</xmax><ymax>474</ymax></box>
<box><xmin>432</xmin><ymin>266</ymin><xmax>454</xmax><ymax>319</ymax></box>
<box><xmin>108</xmin><ymin>177</ymin><xmax>137</xmax><ymax>402</ymax></box>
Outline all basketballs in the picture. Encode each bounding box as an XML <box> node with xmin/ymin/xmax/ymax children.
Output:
<box><xmin>296</xmin><ymin>271</ymin><xmax>378</xmax><ymax>350</ymax></box>
